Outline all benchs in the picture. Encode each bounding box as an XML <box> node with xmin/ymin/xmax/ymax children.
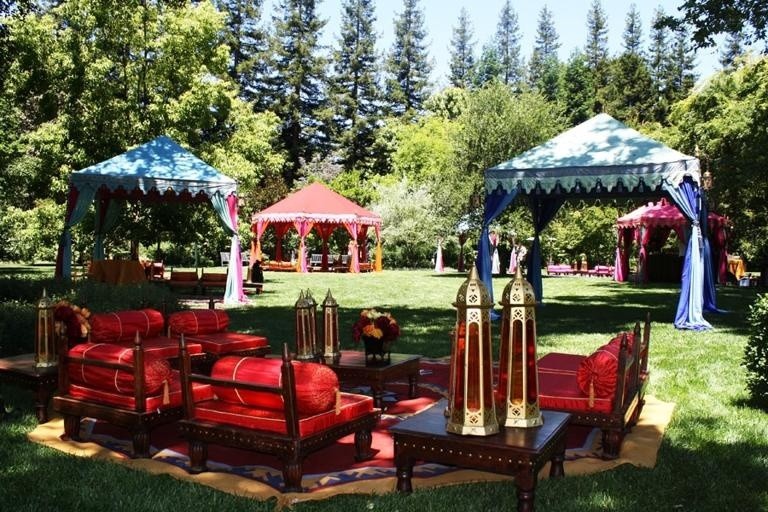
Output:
<box><xmin>169</xmin><ymin>267</ymin><xmax>263</xmax><ymax>294</ymax></box>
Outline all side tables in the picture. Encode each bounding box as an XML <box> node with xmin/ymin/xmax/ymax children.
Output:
<box><xmin>1</xmin><ymin>353</ymin><xmax>58</xmax><ymax>423</ymax></box>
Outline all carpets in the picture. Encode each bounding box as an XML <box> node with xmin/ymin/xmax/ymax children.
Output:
<box><xmin>27</xmin><ymin>392</ymin><xmax>674</xmax><ymax>506</ymax></box>
